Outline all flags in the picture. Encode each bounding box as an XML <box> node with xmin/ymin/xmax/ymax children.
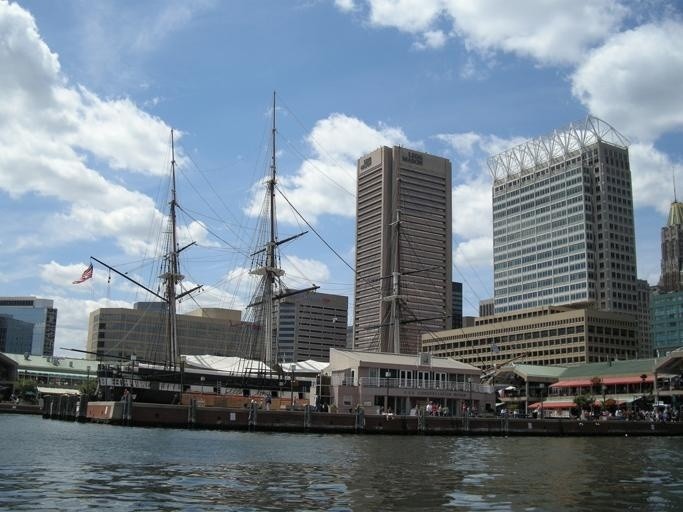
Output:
<box><xmin>71</xmin><ymin>262</ymin><xmax>93</xmax><ymax>284</ymax></box>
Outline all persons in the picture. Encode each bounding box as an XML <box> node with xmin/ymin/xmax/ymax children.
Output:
<box><xmin>615</xmin><ymin>407</ymin><xmax>678</xmax><ymax>422</ymax></box>
<box><xmin>417</xmin><ymin>401</ymin><xmax>449</xmax><ymax>415</ymax></box>
